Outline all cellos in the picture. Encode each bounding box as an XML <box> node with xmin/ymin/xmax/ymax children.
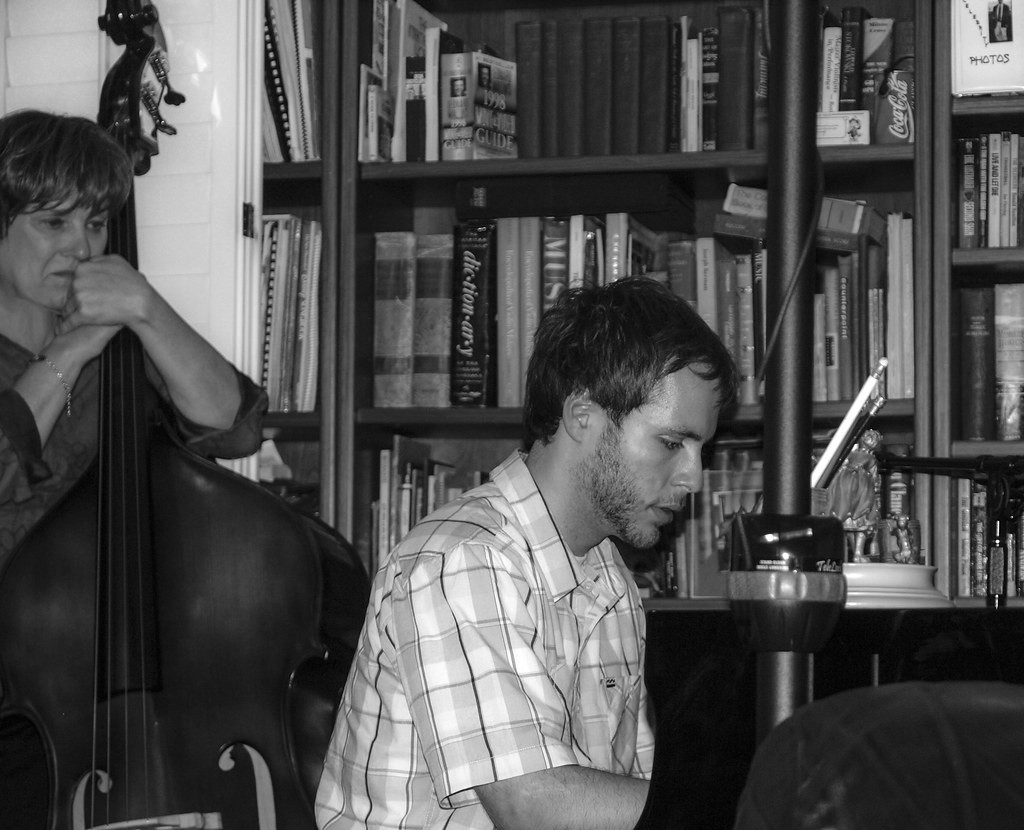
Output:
<box><xmin>0</xmin><ymin>0</ymin><xmax>367</xmax><ymax>829</ymax></box>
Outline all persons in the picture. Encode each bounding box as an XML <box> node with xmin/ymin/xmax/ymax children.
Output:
<box><xmin>313</xmin><ymin>277</ymin><xmax>736</xmax><ymax>830</ymax></box>
<box><xmin>0</xmin><ymin>109</ymin><xmax>270</xmax><ymax>830</ymax></box>
<box><xmin>829</xmin><ymin>431</ymin><xmax>884</xmax><ymax>562</ymax></box>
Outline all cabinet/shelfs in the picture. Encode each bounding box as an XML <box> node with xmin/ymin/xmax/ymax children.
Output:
<box><xmin>261</xmin><ymin>0</ymin><xmax>1023</xmax><ymax>632</ymax></box>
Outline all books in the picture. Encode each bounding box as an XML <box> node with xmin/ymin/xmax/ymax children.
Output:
<box><xmin>258</xmin><ymin>0</ymin><xmax>1024</xmax><ymax>597</ymax></box>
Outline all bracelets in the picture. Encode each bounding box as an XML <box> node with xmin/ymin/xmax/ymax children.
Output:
<box><xmin>31</xmin><ymin>353</ymin><xmax>71</xmax><ymax>416</ymax></box>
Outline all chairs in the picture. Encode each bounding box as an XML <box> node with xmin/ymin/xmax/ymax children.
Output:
<box><xmin>734</xmin><ymin>681</ymin><xmax>1022</xmax><ymax>830</ymax></box>
<box><xmin>280</xmin><ymin>639</ymin><xmax>355</xmax><ymax>826</ymax></box>
